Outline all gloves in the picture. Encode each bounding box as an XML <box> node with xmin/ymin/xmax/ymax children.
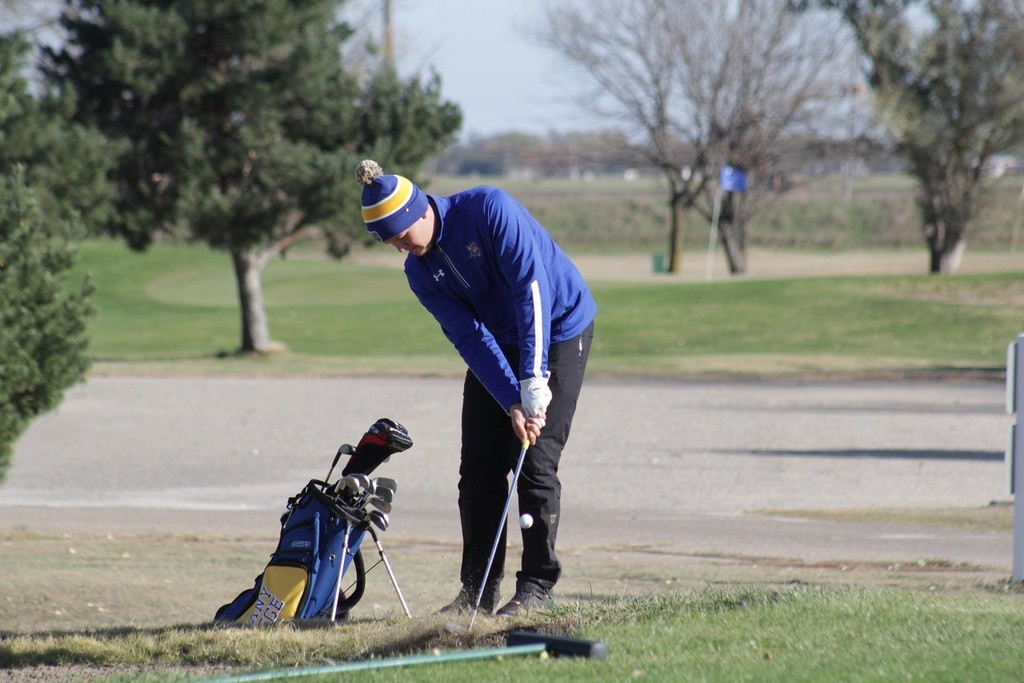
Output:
<box><xmin>521</xmin><ymin>376</ymin><xmax>553</xmax><ymax>417</ymax></box>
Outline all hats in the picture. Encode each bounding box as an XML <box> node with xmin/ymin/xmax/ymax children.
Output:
<box><xmin>355</xmin><ymin>160</ymin><xmax>429</xmax><ymax>240</ymax></box>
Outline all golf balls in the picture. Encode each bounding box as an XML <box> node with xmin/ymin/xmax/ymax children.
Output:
<box><xmin>519</xmin><ymin>514</ymin><xmax>534</xmax><ymax>529</ymax></box>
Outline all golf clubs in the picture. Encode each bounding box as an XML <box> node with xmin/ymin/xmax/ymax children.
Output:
<box><xmin>318</xmin><ymin>443</ymin><xmax>398</xmax><ymax>532</ymax></box>
<box><xmin>445</xmin><ymin>371</ymin><xmax>552</xmax><ymax>635</ymax></box>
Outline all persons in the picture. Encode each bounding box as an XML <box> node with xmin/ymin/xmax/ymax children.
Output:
<box><xmin>361</xmin><ymin>155</ymin><xmax>597</xmax><ymax>616</ymax></box>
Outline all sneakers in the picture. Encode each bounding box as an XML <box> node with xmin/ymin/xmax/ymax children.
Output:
<box><xmin>434</xmin><ymin>576</ymin><xmax>500</xmax><ymax>615</ymax></box>
<box><xmin>497</xmin><ymin>578</ymin><xmax>556</xmax><ymax>617</ymax></box>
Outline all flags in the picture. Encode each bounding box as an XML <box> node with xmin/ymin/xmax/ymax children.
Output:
<box><xmin>719</xmin><ymin>162</ymin><xmax>747</xmax><ymax>193</ymax></box>
